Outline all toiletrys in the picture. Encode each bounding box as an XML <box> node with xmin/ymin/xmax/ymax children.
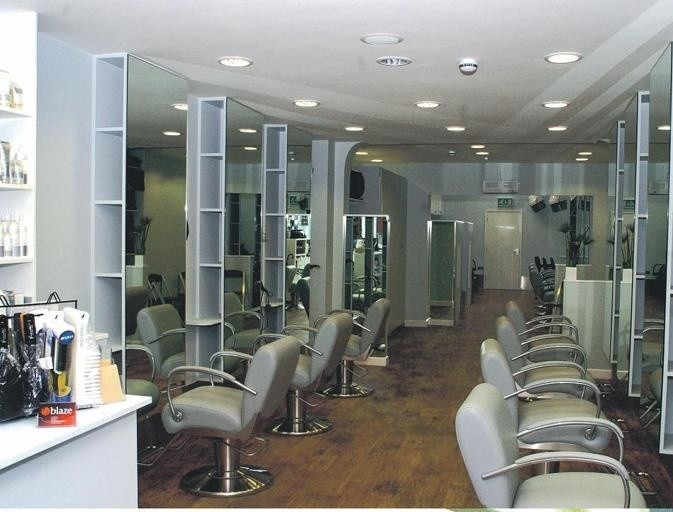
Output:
<box><xmin>0</xmin><ymin>68</ymin><xmax>28</xmax><ymax>259</ymax></box>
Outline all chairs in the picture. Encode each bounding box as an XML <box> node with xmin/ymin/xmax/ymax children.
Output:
<box><xmin>144</xmin><ymin>272</ymin><xmax>174</xmax><ymax>306</ymax></box>
<box><xmin>161</xmin><ymin>333</ymin><xmax>298</xmax><ymax>497</ymax></box>
<box><xmin>454</xmin><ymin>300</ymin><xmax>646</xmax><ymax>506</ymax></box>
<box><xmin>285</xmin><ymin>263</ymin><xmax>297</xmax><ymax>295</ymax></box>
<box><xmin>136</xmin><ymin>303</ymin><xmax>240</xmax><ymax>390</ymax></box>
<box><xmin>313</xmin><ymin>299</ymin><xmax>390</xmax><ymax>398</ymax></box>
<box><xmin>217</xmin><ymin>290</ymin><xmax>273</xmax><ymax>352</ymax></box>
<box><xmin>527</xmin><ymin>255</ymin><xmax>567</xmax><ymax>325</ymax></box>
<box><xmin>124</xmin><ymin>342</ymin><xmax>181</xmax><ymax>466</ymax></box>
<box><xmin>224</xmin><ymin>268</ymin><xmax>247</xmax><ymax>311</ymax></box>
<box><xmin>251</xmin><ymin>312</ymin><xmax>354</xmax><ymax>437</ymax></box>
<box><xmin>177</xmin><ymin>270</ymin><xmax>186</xmax><ymax>295</ymax></box>
<box><xmin>296</xmin><ymin>276</ymin><xmax>311</xmax><ymax>314</ymax></box>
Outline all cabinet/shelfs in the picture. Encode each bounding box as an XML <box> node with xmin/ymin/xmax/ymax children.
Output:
<box><xmin>0</xmin><ymin>105</ymin><xmax>33</xmax><ymax>265</ymax></box>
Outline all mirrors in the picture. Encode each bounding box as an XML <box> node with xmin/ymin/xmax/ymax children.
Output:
<box><xmin>611</xmin><ymin>42</ymin><xmax>671</xmax><ymax>453</ymax></box>
<box><xmin>122</xmin><ymin>54</ymin><xmax>313</xmax><ymax>456</ymax></box>
